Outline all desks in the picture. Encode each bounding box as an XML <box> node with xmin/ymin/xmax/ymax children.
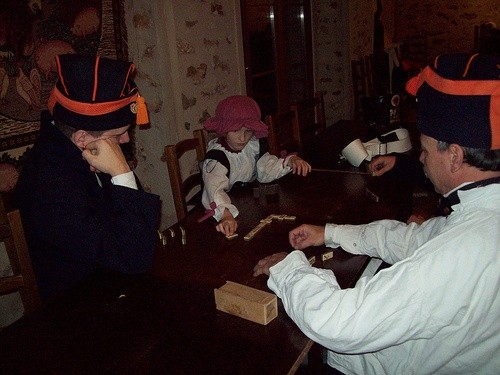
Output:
<box><xmin>0</xmin><ymin>160</ymin><xmax>434</xmax><ymax>375</ymax></box>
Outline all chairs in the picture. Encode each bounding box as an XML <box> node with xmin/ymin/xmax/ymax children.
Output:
<box><xmin>266</xmin><ymin>91</ymin><xmax>326</xmax><ymax>157</ymax></box>
<box><xmin>0</xmin><ymin>209</ymin><xmax>40</xmax><ymax>316</ymax></box>
<box><xmin>160</xmin><ymin>130</ymin><xmax>206</xmax><ymax>221</ymax></box>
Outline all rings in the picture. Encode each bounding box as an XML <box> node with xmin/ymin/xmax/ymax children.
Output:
<box><xmin>257</xmin><ymin>259</ymin><xmax>267</xmax><ymax>266</ymax></box>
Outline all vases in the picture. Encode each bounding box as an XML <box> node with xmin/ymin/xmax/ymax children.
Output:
<box><xmin>361</xmin><ymin>94</ymin><xmax>401</xmax><ymax>124</ymax></box>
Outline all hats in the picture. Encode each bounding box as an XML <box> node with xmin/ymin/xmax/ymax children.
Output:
<box><xmin>205</xmin><ymin>95</ymin><xmax>270</xmax><ymax>138</ymax></box>
<box><xmin>47</xmin><ymin>53</ymin><xmax>151</xmax><ymax>130</ymax></box>
<box><xmin>405</xmin><ymin>51</ymin><xmax>500</xmax><ymax>152</ymax></box>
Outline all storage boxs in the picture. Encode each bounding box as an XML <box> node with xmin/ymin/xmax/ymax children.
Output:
<box><xmin>214</xmin><ymin>280</ymin><xmax>278</xmax><ymax>325</ymax></box>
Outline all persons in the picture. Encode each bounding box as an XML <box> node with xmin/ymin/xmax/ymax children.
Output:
<box><xmin>369</xmin><ymin>151</ymin><xmax>453</xmax><ymax>225</ymax></box>
<box><xmin>13</xmin><ymin>53</ymin><xmax>163</xmax><ymax>307</ymax></box>
<box><xmin>201</xmin><ymin>95</ymin><xmax>311</xmax><ymax>238</ymax></box>
<box><xmin>253</xmin><ymin>52</ymin><xmax>500</xmax><ymax>375</ymax></box>
